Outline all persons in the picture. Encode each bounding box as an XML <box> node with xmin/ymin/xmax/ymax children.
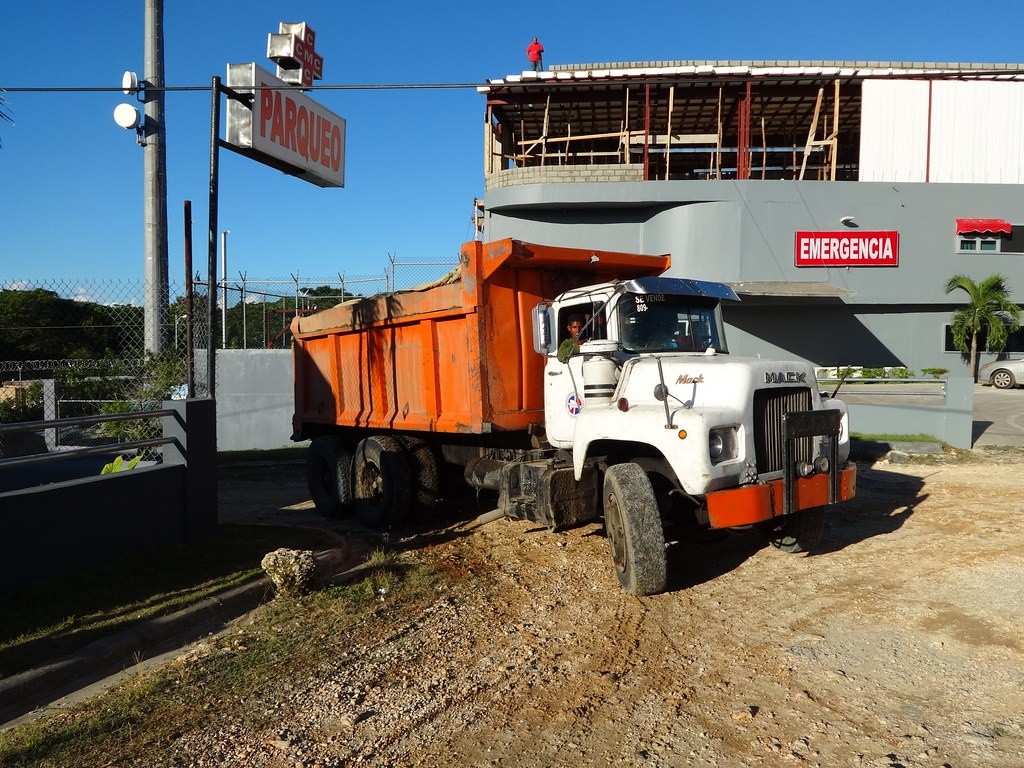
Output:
<box><xmin>526</xmin><ymin>37</ymin><xmax>544</xmax><ymax>71</ymax></box>
<box><xmin>557</xmin><ymin>314</ymin><xmax>588</xmax><ymax>364</ymax></box>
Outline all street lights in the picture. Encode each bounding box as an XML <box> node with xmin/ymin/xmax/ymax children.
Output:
<box><xmin>175</xmin><ymin>314</ymin><xmax>187</xmax><ymax>350</ymax></box>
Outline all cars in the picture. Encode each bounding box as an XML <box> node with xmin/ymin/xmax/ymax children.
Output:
<box><xmin>979</xmin><ymin>358</ymin><xmax>1023</xmax><ymax>390</ymax></box>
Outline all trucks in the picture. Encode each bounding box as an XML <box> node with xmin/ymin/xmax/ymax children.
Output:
<box><xmin>290</xmin><ymin>236</ymin><xmax>858</xmax><ymax>595</ymax></box>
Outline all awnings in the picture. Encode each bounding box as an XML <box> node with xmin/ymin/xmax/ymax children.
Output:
<box><xmin>956</xmin><ymin>218</ymin><xmax>1012</xmax><ymax>234</ymax></box>
